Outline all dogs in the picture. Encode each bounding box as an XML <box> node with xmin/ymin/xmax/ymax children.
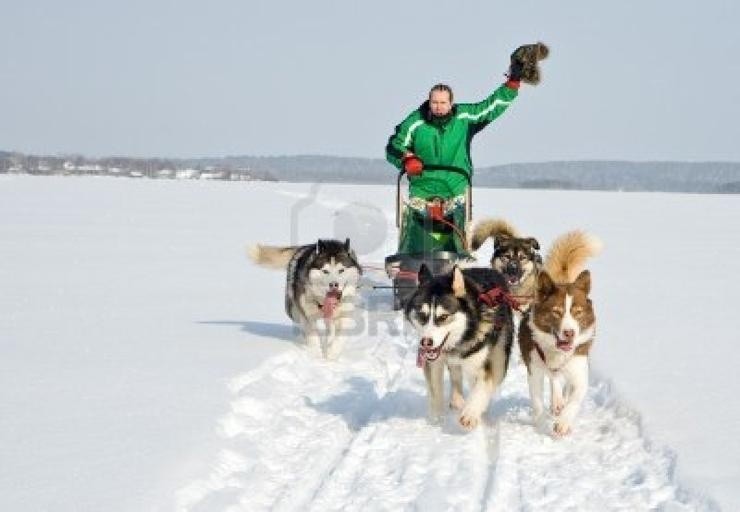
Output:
<box><xmin>515</xmin><ymin>229</ymin><xmax>605</xmax><ymax>438</ymax></box>
<box><xmin>249</xmin><ymin>237</ymin><xmax>362</xmax><ymax>358</ymax></box>
<box><xmin>470</xmin><ymin>217</ymin><xmax>544</xmax><ymax>309</ymax></box>
<box><xmin>400</xmin><ymin>262</ymin><xmax>514</xmax><ymax>430</ymax></box>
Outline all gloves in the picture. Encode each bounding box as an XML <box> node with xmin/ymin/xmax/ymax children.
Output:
<box><xmin>404</xmin><ymin>153</ymin><xmax>422</xmax><ymax>176</ymax></box>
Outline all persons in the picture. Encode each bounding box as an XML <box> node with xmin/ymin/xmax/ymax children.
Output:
<box><xmin>386</xmin><ymin>66</ymin><xmax>526</xmax><ymax>254</ymax></box>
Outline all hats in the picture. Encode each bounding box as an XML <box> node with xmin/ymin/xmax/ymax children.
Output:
<box><xmin>511</xmin><ymin>43</ymin><xmax>548</xmax><ymax>83</ymax></box>
